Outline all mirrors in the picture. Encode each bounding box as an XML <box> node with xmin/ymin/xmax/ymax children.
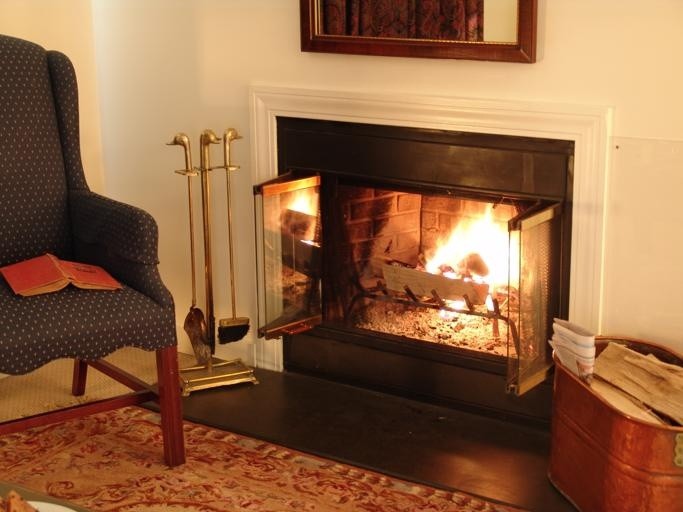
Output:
<box><xmin>296</xmin><ymin>1</ymin><xmax>537</xmax><ymax>66</ymax></box>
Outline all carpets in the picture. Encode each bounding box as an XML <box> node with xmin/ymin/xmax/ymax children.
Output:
<box><xmin>0</xmin><ymin>394</ymin><xmax>533</xmax><ymax>511</ymax></box>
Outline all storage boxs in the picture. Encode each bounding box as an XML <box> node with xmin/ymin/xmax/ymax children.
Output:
<box><xmin>547</xmin><ymin>333</ymin><xmax>682</xmax><ymax>512</ymax></box>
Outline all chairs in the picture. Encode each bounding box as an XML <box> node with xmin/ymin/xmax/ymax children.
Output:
<box><xmin>0</xmin><ymin>35</ymin><xmax>188</xmax><ymax>471</ymax></box>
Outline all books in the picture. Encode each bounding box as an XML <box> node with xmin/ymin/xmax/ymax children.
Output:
<box><xmin>0</xmin><ymin>252</ymin><xmax>123</xmax><ymax>298</ymax></box>
<box><xmin>592</xmin><ymin>341</ymin><xmax>683</xmax><ymax>427</ymax></box>
<box><xmin>588</xmin><ymin>375</ymin><xmax>671</xmax><ymax>427</ymax></box>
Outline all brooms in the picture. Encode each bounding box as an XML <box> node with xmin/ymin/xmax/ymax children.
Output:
<box><xmin>217</xmin><ymin>128</ymin><xmax>250</xmax><ymax>346</ymax></box>
<box><xmin>165</xmin><ymin>133</ymin><xmax>210</xmax><ymax>366</ymax></box>
